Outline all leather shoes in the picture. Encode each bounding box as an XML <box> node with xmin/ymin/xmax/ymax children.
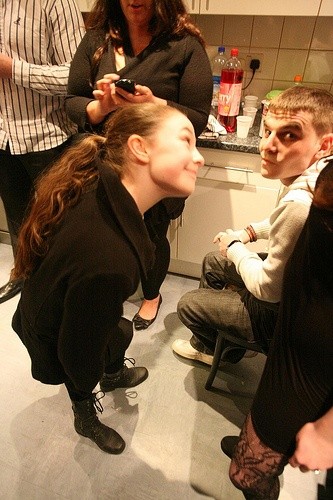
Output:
<box><xmin>0</xmin><ymin>279</ymin><xmax>23</xmax><ymax>304</ymax></box>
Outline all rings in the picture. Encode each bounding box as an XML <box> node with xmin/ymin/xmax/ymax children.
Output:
<box><xmin>312</xmin><ymin>469</ymin><xmax>320</xmax><ymax>475</ymax></box>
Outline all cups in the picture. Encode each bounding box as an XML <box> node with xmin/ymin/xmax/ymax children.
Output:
<box><xmin>236</xmin><ymin>116</ymin><xmax>252</xmax><ymax>138</ymax></box>
<box><xmin>259</xmin><ymin>99</ymin><xmax>271</xmax><ymax>138</ymax></box>
<box><xmin>245</xmin><ymin>95</ymin><xmax>259</xmax><ymax>108</ymax></box>
<box><xmin>242</xmin><ymin>107</ymin><xmax>258</xmax><ymax>129</ymax></box>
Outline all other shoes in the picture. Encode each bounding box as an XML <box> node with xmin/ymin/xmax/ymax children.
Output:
<box><xmin>218</xmin><ymin>344</ymin><xmax>246</xmax><ymax>368</ymax></box>
<box><xmin>132</xmin><ymin>294</ymin><xmax>162</xmax><ymax>331</ymax></box>
<box><xmin>221</xmin><ymin>435</ymin><xmax>239</xmax><ymax>457</ymax></box>
<box><xmin>172</xmin><ymin>338</ymin><xmax>214</xmax><ymax>366</ymax></box>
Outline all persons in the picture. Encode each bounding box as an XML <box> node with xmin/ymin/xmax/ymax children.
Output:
<box><xmin>0</xmin><ymin>1</ymin><xmax>87</xmax><ymax>305</ymax></box>
<box><xmin>10</xmin><ymin>103</ymin><xmax>206</xmax><ymax>454</ymax></box>
<box><xmin>190</xmin><ymin>159</ymin><xmax>333</xmax><ymax>500</ymax></box>
<box><xmin>63</xmin><ymin>0</ymin><xmax>214</xmax><ymax>331</ymax></box>
<box><xmin>171</xmin><ymin>85</ymin><xmax>333</xmax><ymax>366</ymax></box>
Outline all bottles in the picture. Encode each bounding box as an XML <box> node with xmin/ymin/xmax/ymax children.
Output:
<box><xmin>210</xmin><ymin>46</ymin><xmax>228</xmax><ymax>118</ymax></box>
<box><xmin>217</xmin><ymin>47</ymin><xmax>245</xmax><ymax>133</ymax></box>
<box><xmin>292</xmin><ymin>75</ymin><xmax>302</xmax><ymax>86</ymax></box>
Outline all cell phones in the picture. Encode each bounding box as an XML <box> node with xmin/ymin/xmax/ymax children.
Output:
<box><xmin>115</xmin><ymin>79</ymin><xmax>135</xmax><ymax>99</ymax></box>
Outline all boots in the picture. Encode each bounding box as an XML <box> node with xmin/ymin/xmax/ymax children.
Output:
<box><xmin>71</xmin><ymin>395</ymin><xmax>126</xmax><ymax>454</ymax></box>
<box><xmin>100</xmin><ymin>362</ymin><xmax>148</xmax><ymax>392</ymax></box>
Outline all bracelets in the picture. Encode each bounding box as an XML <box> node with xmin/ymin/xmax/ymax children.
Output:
<box><xmin>225</xmin><ymin>239</ymin><xmax>241</xmax><ymax>252</ymax></box>
<box><xmin>244</xmin><ymin>226</ymin><xmax>257</xmax><ymax>242</ymax></box>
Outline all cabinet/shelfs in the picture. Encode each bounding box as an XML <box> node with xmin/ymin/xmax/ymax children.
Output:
<box><xmin>75</xmin><ymin>0</ymin><xmax>333</xmax><ymax>17</ymax></box>
<box><xmin>165</xmin><ymin>145</ymin><xmax>289</xmax><ymax>279</ymax></box>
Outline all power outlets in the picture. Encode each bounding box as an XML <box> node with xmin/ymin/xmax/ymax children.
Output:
<box><xmin>207</xmin><ymin>51</ymin><xmax>264</xmax><ymax>73</ymax></box>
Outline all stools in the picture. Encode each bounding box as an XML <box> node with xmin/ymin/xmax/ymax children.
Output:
<box><xmin>204</xmin><ymin>327</ymin><xmax>273</xmax><ymax>390</ymax></box>
